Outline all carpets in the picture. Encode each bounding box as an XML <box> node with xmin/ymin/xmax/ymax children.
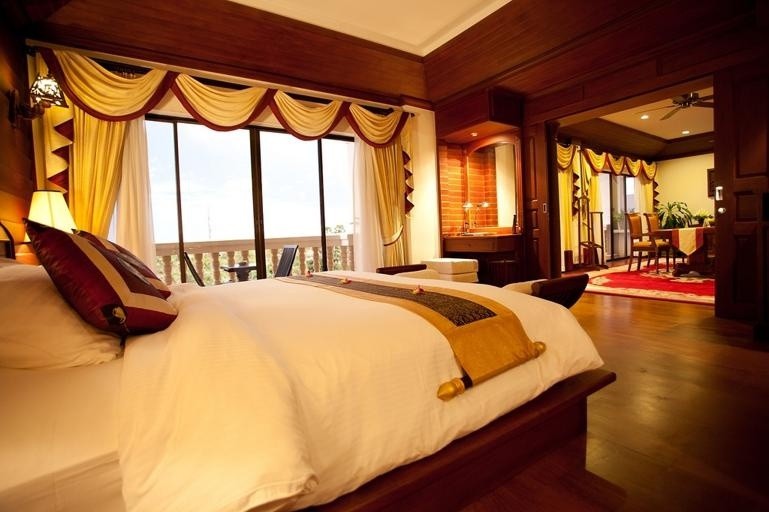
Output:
<box><xmin>584</xmin><ymin>257</ymin><xmax>716</xmax><ymax>304</ymax></box>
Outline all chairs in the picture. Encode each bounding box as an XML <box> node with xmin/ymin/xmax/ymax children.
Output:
<box><xmin>626</xmin><ymin>214</ymin><xmax>657</xmax><ymax>273</ymax></box>
<box><xmin>644</xmin><ymin>212</ymin><xmax>675</xmax><ymax>268</ymax></box>
<box><xmin>271</xmin><ymin>244</ymin><xmax>299</xmax><ymax>278</ymax></box>
<box><xmin>182</xmin><ymin>250</ymin><xmax>203</xmax><ymax>287</ymax></box>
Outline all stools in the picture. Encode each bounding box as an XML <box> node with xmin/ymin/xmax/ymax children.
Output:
<box><xmin>421</xmin><ymin>258</ymin><xmax>480</xmax><ymax>283</ymax></box>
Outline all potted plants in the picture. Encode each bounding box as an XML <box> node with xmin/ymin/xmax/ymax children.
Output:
<box><xmin>690</xmin><ymin>209</ymin><xmax>714</xmax><ymax>228</ymax></box>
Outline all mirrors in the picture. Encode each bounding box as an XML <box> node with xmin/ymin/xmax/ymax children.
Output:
<box><xmin>468</xmin><ymin>142</ymin><xmax>516</xmax><ymax>229</ymax></box>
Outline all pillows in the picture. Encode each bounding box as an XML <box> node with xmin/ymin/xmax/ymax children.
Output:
<box><xmin>71</xmin><ymin>227</ymin><xmax>171</xmax><ymax>302</ymax></box>
<box><xmin>501</xmin><ymin>278</ymin><xmax>549</xmax><ymax>296</ymax></box>
<box><xmin>394</xmin><ymin>268</ymin><xmax>440</xmax><ymax>282</ymax></box>
<box><xmin>24</xmin><ymin>219</ymin><xmax>178</xmax><ymax>339</ymax></box>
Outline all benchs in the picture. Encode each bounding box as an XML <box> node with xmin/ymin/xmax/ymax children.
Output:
<box><xmin>376</xmin><ymin>264</ymin><xmax>589</xmax><ymax>312</ymax></box>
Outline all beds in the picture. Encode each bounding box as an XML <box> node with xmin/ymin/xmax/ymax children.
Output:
<box><xmin>0</xmin><ymin>216</ymin><xmax>616</xmax><ymax>509</ymax></box>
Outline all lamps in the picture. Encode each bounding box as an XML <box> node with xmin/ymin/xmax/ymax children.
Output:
<box><xmin>24</xmin><ymin>189</ymin><xmax>80</xmax><ymax>241</ymax></box>
<box><xmin>9</xmin><ymin>72</ymin><xmax>69</xmax><ymax>124</ymax></box>
<box><xmin>463</xmin><ymin>202</ymin><xmax>473</xmax><ymax>236</ymax></box>
<box><xmin>473</xmin><ymin>201</ymin><xmax>489</xmax><ymax>230</ymax></box>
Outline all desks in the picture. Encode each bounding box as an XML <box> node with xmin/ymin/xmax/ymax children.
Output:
<box><xmin>444</xmin><ymin>234</ymin><xmax>519</xmax><ymax>284</ymax></box>
<box><xmin>218</xmin><ymin>264</ymin><xmax>260</xmax><ymax>285</ymax></box>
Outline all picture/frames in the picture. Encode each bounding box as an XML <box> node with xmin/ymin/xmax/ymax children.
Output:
<box><xmin>707</xmin><ymin>168</ymin><xmax>716</xmax><ymax>198</ymax></box>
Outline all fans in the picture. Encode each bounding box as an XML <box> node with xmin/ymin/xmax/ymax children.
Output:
<box><xmin>634</xmin><ymin>91</ymin><xmax>715</xmax><ymax>122</ymax></box>
